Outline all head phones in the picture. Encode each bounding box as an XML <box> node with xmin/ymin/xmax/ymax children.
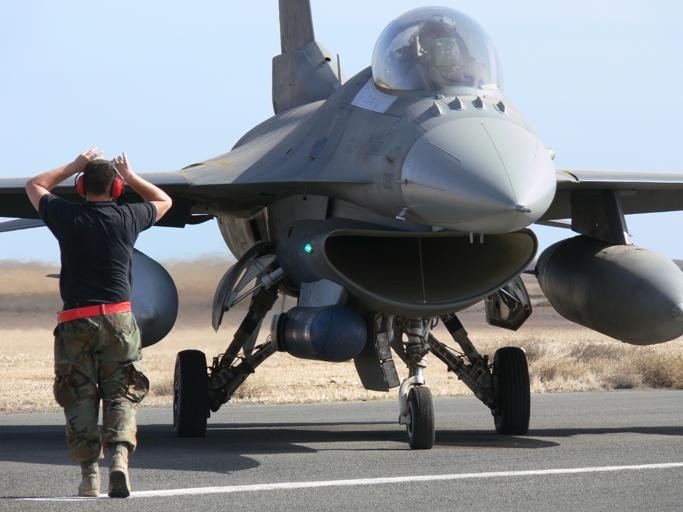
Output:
<box><xmin>74</xmin><ymin>167</ymin><xmax>125</xmax><ymax>198</ymax></box>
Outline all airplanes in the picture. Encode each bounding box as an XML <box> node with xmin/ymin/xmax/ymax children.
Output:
<box><xmin>0</xmin><ymin>1</ymin><xmax>682</xmax><ymax>449</ymax></box>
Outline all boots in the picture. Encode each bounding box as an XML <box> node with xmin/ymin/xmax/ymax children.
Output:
<box><xmin>78</xmin><ymin>461</ymin><xmax>101</xmax><ymax>497</ymax></box>
<box><xmin>108</xmin><ymin>442</ymin><xmax>131</xmax><ymax>497</ymax></box>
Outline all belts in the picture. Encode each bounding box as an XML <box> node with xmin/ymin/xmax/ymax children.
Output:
<box><xmin>57</xmin><ymin>301</ymin><xmax>130</xmax><ymax>320</ymax></box>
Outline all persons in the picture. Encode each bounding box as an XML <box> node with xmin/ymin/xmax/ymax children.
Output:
<box><xmin>24</xmin><ymin>147</ymin><xmax>173</xmax><ymax>498</ymax></box>
